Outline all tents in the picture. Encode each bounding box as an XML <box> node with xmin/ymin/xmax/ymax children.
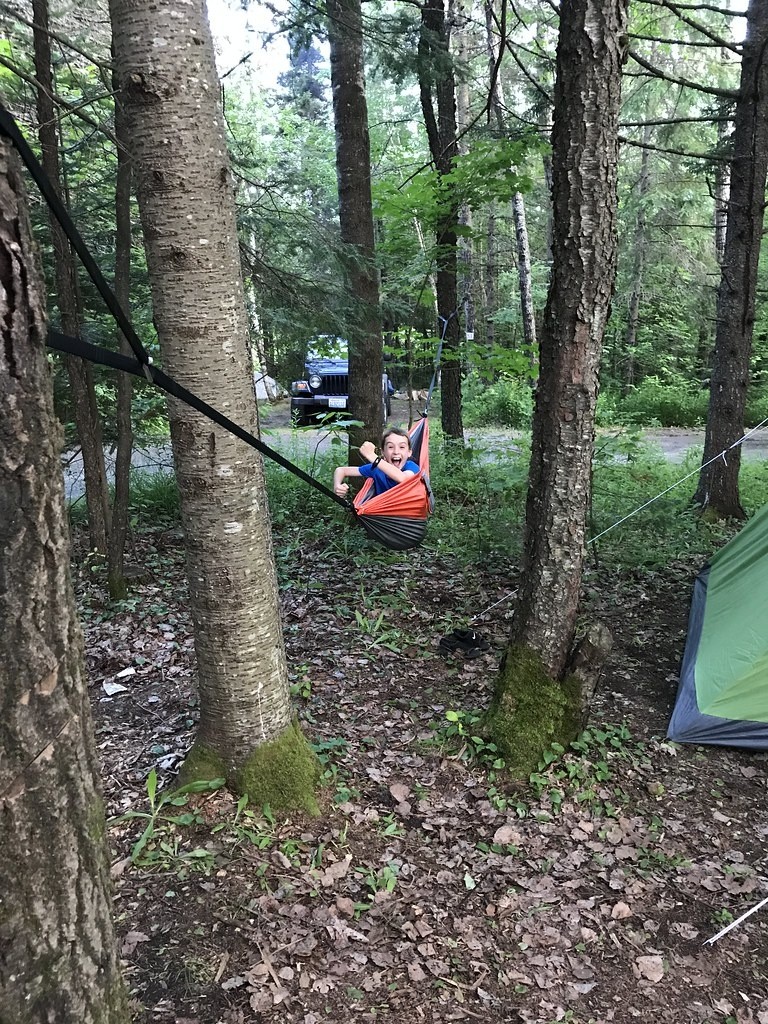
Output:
<box><xmin>666</xmin><ymin>502</ymin><xmax>768</xmax><ymax>748</ymax></box>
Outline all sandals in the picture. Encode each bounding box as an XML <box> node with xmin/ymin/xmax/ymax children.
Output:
<box><xmin>449</xmin><ymin>629</ymin><xmax>489</xmax><ymax>652</ymax></box>
<box><xmin>440</xmin><ymin>638</ymin><xmax>480</xmax><ymax>659</ymax></box>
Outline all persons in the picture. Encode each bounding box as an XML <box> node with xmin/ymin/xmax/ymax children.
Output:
<box><xmin>334</xmin><ymin>427</ymin><xmax>420</xmax><ymax>499</ymax></box>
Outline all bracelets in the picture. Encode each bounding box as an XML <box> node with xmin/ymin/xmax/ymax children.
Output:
<box><xmin>371</xmin><ymin>457</ymin><xmax>381</xmax><ymax>471</ymax></box>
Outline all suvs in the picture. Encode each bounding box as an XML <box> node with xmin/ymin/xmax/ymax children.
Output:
<box><xmin>288</xmin><ymin>334</ymin><xmax>395</xmax><ymax>428</ymax></box>
